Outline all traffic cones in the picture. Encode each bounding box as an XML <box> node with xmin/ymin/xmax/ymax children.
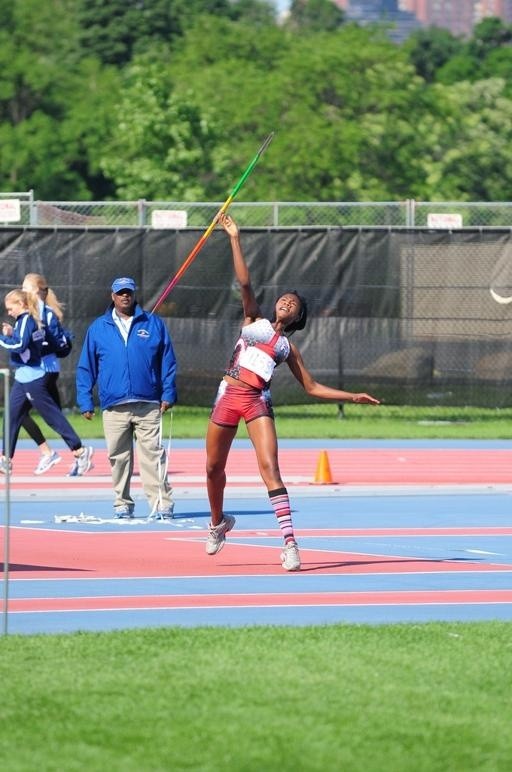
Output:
<box><xmin>304</xmin><ymin>447</ymin><xmax>341</xmax><ymax>487</ymax></box>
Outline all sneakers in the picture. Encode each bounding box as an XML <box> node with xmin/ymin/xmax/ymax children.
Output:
<box><xmin>204</xmin><ymin>515</ymin><xmax>236</xmax><ymax>555</ymax></box>
<box><xmin>69</xmin><ymin>443</ymin><xmax>95</xmax><ymax>478</ymax></box>
<box><xmin>114</xmin><ymin>503</ymin><xmax>134</xmax><ymax>520</ymax></box>
<box><xmin>280</xmin><ymin>545</ymin><xmax>302</xmax><ymax>571</ymax></box>
<box><xmin>155</xmin><ymin>507</ymin><xmax>174</xmax><ymax>521</ymax></box>
<box><xmin>33</xmin><ymin>451</ymin><xmax>61</xmax><ymax>475</ymax></box>
<box><xmin>0</xmin><ymin>456</ymin><xmax>13</xmax><ymax>476</ymax></box>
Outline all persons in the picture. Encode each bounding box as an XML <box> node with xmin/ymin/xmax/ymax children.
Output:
<box><xmin>203</xmin><ymin>212</ymin><xmax>383</xmax><ymax>573</ymax></box>
<box><xmin>17</xmin><ymin>270</ymin><xmax>65</xmax><ymax>476</ymax></box>
<box><xmin>0</xmin><ymin>288</ymin><xmax>94</xmax><ymax>479</ymax></box>
<box><xmin>74</xmin><ymin>276</ymin><xmax>179</xmax><ymax>522</ymax></box>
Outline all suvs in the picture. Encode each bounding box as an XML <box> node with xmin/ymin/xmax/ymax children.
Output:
<box><xmin>425</xmin><ymin>368</ymin><xmax>486</xmax><ymax>407</ymax></box>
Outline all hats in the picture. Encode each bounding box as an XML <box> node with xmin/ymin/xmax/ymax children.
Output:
<box><xmin>111</xmin><ymin>276</ymin><xmax>136</xmax><ymax>294</ymax></box>
